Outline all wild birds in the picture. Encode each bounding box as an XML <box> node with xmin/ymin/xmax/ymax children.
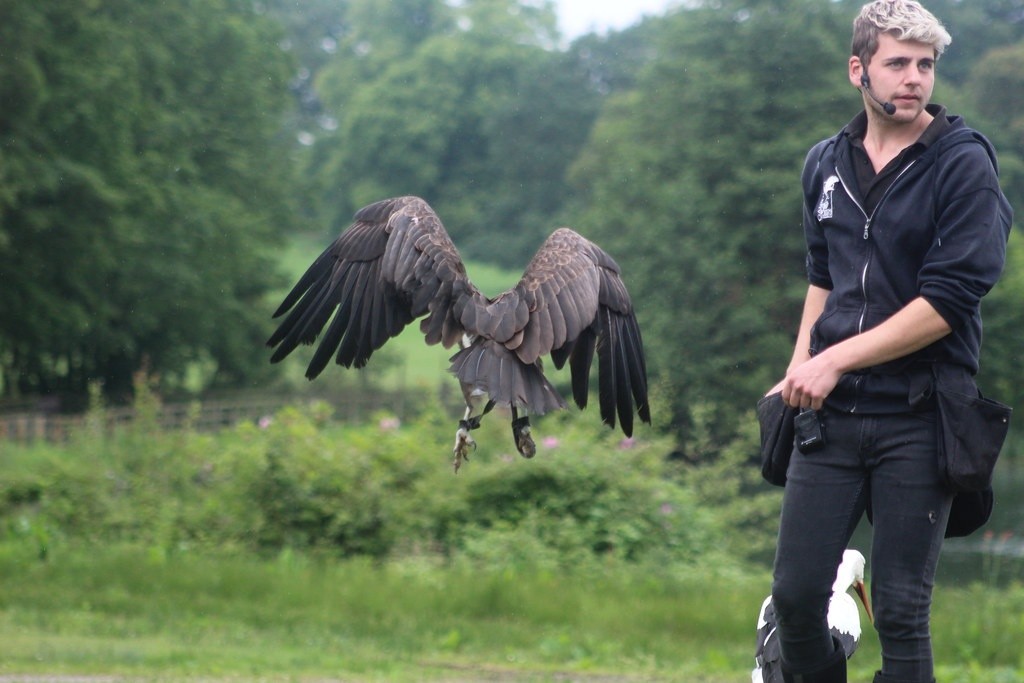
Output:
<box><xmin>751</xmin><ymin>548</ymin><xmax>872</xmax><ymax>683</ymax></box>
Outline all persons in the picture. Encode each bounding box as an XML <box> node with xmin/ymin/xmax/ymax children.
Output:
<box><xmin>771</xmin><ymin>0</ymin><xmax>1014</xmax><ymax>682</ymax></box>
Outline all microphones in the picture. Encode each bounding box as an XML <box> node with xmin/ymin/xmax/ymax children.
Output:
<box><xmin>864</xmin><ymin>80</ymin><xmax>897</xmax><ymax>114</ymax></box>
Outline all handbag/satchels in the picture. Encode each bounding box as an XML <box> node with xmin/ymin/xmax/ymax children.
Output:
<box><xmin>757</xmin><ymin>389</ymin><xmax>801</xmax><ymax>489</ymax></box>
<box><xmin>935</xmin><ymin>386</ymin><xmax>1013</xmax><ymax>496</ymax></box>
